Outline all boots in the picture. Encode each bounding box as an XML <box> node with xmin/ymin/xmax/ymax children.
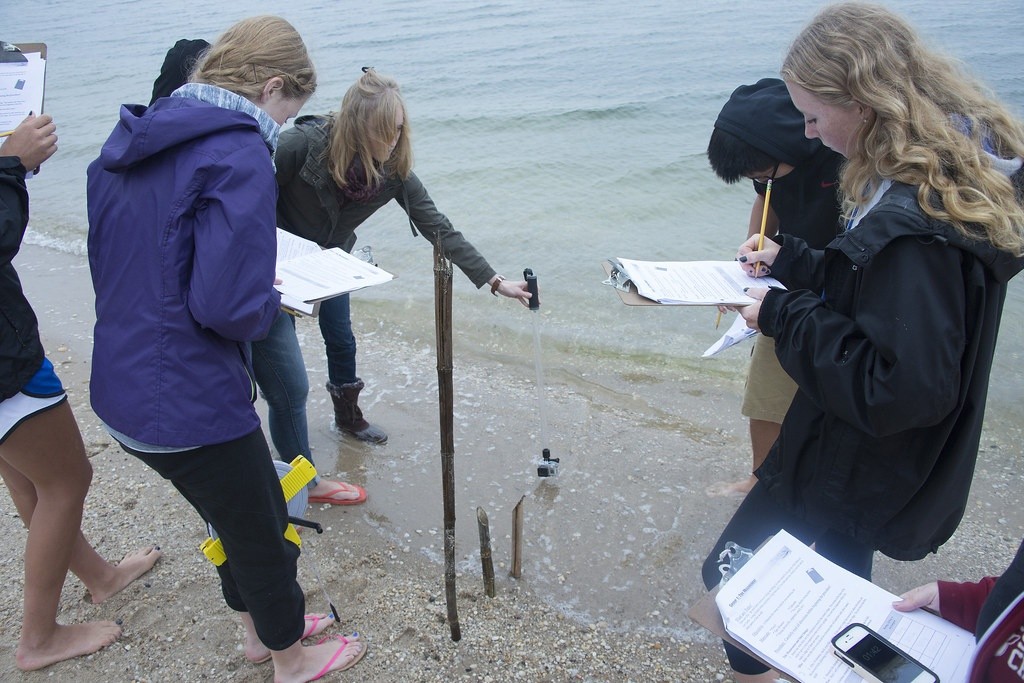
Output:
<box><xmin>326</xmin><ymin>377</ymin><xmax>387</xmax><ymax>443</ymax></box>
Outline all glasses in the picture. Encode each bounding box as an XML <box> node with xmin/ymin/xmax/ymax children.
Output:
<box><xmin>754</xmin><ymin>162</ymin><xmax>779</xmax><ymax>185</ymax></box>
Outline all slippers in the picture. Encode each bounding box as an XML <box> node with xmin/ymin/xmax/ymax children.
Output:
<box><xmin>252</xmin><ymin>615</ymin><xmax>334</xmax><ymax>664</ymax></box>
<box><xmin>308</xmin><ymin>482</ymin><xmax>366</xmax><ymax>505</ymax></box>
<box><xmin>311</xmin><ymin>634</ymin><xmax>367</xmax><ymax>681</ymax></box>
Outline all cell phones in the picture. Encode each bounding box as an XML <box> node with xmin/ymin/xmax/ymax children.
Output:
<box><xmin>829</xmin><ymin>622</ymin><xmax>941</xmax><ymax>683</ymax></box>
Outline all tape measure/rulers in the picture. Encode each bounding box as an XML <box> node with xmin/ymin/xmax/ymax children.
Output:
<box><xmin>197</xmin><ymin>451</ymin><xmax>324</xmax><ymax>568</ymax></box>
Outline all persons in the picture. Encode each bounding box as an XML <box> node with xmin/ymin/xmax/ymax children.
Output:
<box><xmin>707</xmin><ymin>78</ymin><xmax>853</xmax><ymax>492</ymax></box>
<box><xmin>701</xmin><ymin>1</ymin><xmax>1024</xmax><ymax>683</ymax></box>
<box><xmin>278</xmin><ymin>67</ymin><xmax>542</xmax><ymax>444</ymax></box>
<box><xmin>892</xmin><ymin>543</ymin><xmax>1024</xmax><ymax>681</ymax></box>
<box><xmin>137</xmin><ymin>37</ymin><xmax>213</xmax><ymax>106</ymax></box>
<box><xmin>87</xmin><ymin>16</ymin><xmax>368</xmax><ymax>683</ymax></box>
<box><xmin>0</xmin><ymin>110</ymin><xmax>165</xmax><ymax>673</ymax></box>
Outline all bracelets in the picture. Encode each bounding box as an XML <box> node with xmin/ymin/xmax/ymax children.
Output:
<box><xmin>490</xmin><ymin>275</ymin><xmax>506</xmax><ymax>298</ymax></box>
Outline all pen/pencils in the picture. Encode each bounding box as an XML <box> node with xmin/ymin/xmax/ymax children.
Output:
<box><xmin>715</xmin><ymin>310</ymin><xmax>723</xmax><ymax>331</ymax></box>
<box><xmin>279</xmin><ymin>305</ymin><xmax>304</xmax><ymax>320</ymax></box>
<box><xmin>753</xmin><ymin>178</ymin><xmax>773</xmax><ymax>280</ymax></box>
<box><xmin>0</xmin><ymin>129</ymin><xmax>14</xmax><ymax>138</ymax></box>
